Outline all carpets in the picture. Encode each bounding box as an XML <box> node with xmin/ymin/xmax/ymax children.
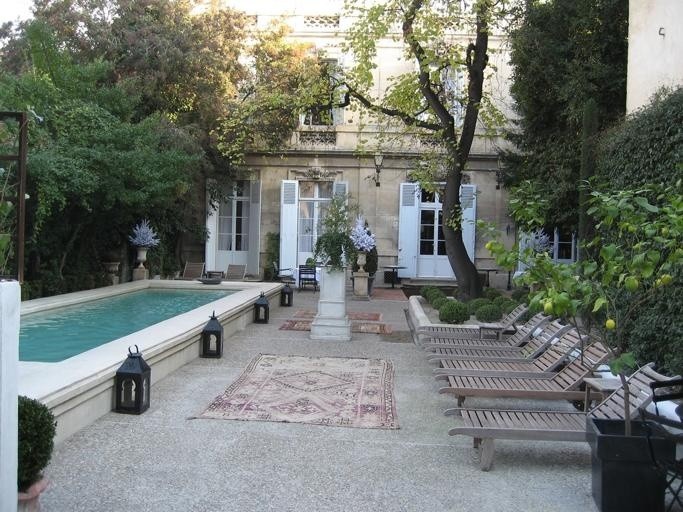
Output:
<box><xmin>188</xmin><ymin>353</ymin><xmax>402</xmax><ymax>432</ymax></box>
<box><xmin>278</xmin><ymin>310</ymin><xmax>388</xmax><ymax>334</ymax></box>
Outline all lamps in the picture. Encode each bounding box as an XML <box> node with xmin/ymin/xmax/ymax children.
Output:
<box><xmin>373</xmin><ymin>150</ymin><xmax>384</xmax><ymax>187</ymax></box>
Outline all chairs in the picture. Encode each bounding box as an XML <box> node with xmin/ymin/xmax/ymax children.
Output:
<box><xmin>638</xmin><ymin>380</ymin><xmax>682</xmax><ymax>512</ymax></box>
<box><xmin>439</xmin><ymin>340</ymin><xmax>623</xmax><ymax>418</ymax></box>
<box><xmin>273</xmin><ymin>261</ymin><xmax>318</xmax><ymax>293</ymax></box>
<box><xmin>422</xmin><ymin>312</ymin><xmax>554</xmax><ymax>359</ymax></box>
<box><xmin>430</xmin><ymin>326</ymin><xmax>590</xmax><ymax>381</ymax></box>
<box><xmin>443</xmin><ymin>361</ymin><xmax>681</xmax><ymax>472</ymax></box>
<box><xmin>418</xmin><ymin>304</ymin><xmax>531</xmax><ymax>348</ymax></box>
<box><xmin>426</xmin><ymin>318</ymin><xmax>571</xmax><ymax>376</ymax></box>
<box><xmin>175</xmin><ymin>260</ymin><xmax>247</xmax><ymax>285</ymax></box>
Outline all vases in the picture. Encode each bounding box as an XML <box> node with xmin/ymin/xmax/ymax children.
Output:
<box><xmin>356</xmin><ymin>251</ymin><xmax>368</xmax><ymax>273</ymax></box>
<box><xmin>134</xmin><ymin>247</ymin><xmax>149</xmax><ymax>269</ymax></box>
<box><xmin>102</xmin><ymin>261</ymin><xmax>121</xmax><ymax>277</ymax></box>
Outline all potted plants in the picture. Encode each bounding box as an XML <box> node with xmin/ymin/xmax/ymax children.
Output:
<box><xmin>473</xmin><ymin>180</ymin><xmax>682</xmax><ymax>512</ymax></box>
<box><xmin>310</xmin><ymin>191</ymin><xmax>361</xmax><ymax>342</ymax></box>
<box><xmin>17</xmin><ymin>393</ymin><xmax>57</xmax><ymax>512</ymax></box>
<box><xmin>347</xmin><ymin>230</ymin><xmax>378</xmax><ymax>296</ymax></box>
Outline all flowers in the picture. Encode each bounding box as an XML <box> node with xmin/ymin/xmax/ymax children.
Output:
<box><xmin>128</xmin><ymin>219</ymin><xmax>161</xmax><ymax>247</ymax></box>
<box><xmin>350</xmin><ymin>214</ymin><xmax>375</xmax><ymax>252</ymax></box>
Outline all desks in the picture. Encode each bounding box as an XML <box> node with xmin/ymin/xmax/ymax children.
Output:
<box><xmin>583</xmin><ymin>376</ymin><xmax>623</xmax><ymax>413</ymax></box>
<box><xmin>380</xmin><ymin>265</ymin><xmax>408</xmax><ymax>288</ymax></box>
